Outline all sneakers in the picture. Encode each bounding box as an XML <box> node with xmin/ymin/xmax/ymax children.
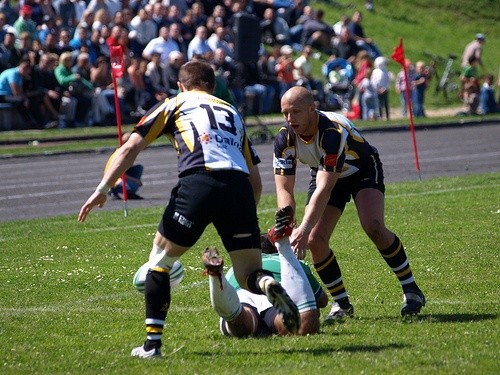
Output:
<box><xmin>267</xmin><ymin>205</ymin><xmax>296</xmax><ymax>246</ymax></box>
<box><xmin>324</xmin><ymin>302</ymin><xmax>354</xmax><ymax>325</ymax></box>
<box><xmin>266</xmin><ymin>281</ymin><xmax>299</xmax><ymax>334</ymax></box>
<box><xmin>401</xmin><ymin>289</ymin><xmax>425</xmax><ymax>316</ymax></box>
<box><xmin>202</xmin><ymin>246</ymin><xmax>223</xmax><ymax>276</ymax></box>
<box><xmin>131</xmin><ymin>344</ymin><xmax>161</xmax><ymax>359</ymax></box>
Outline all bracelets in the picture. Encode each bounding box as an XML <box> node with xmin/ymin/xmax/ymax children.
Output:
<box><xmin>94</xmin><ymin>181</ymin><xmax>111</xmax><ymax>195</ymax></box>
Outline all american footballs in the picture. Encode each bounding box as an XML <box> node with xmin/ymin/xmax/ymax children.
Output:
<box><xmin>133</xmin><ymin>259</ymin><xmax>185</xmax><ymax>294</ymax></box>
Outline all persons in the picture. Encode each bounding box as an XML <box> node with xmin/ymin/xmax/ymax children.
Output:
<box><xmin>0</xmin><ymin>0</ymin><xmax>387</xmax><ymax>127</ymax></box>
<box><xmin>273</xmin><ymin>86</ymin><xmax>425</xmax><ymax>327</ymax></box>
<box><xmin>461</xmin><ymin>56</ymin><xmax>500</xmax><ymax>115</ymax></box>
<box><xmin>202</xmin><ymin>222</ymin><xmax>328</xmax><ymax>339</ymax></box>
<box><xmin>396</xmin><ymin>59</ymin><xmax>428</xmax><ymax>117</ymax></box>
<box><xmin>461</xmin><ymin>33</ymin><xmax>486</xmax><ymax>76</ymax></box>
<box><xmin>78</xmin><ymin>62</ymin><xmax>264</xmax><ymax>359</ymax></box>
